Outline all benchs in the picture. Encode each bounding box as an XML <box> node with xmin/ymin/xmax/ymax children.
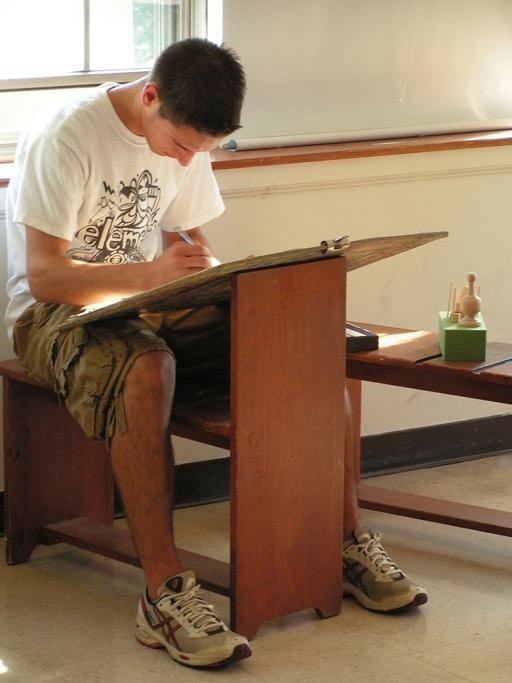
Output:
<box><xmin>345</xmin><ymin>320</ymin><xmax>512</xmax><ymax>539</ymax></box>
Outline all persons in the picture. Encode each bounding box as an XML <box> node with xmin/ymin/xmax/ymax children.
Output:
<box><xmin>1</xmin><ymin>36</ymin><xmax>430</xmax><ymax>671</ymax></box>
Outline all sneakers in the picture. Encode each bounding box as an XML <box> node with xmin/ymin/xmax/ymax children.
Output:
<box><xmin>341</xmin><ymin>525</ymin><xmax>428</xmax><ymax>613</ymax></box>
<box><xmin>137</xmin><ymin>570</ymin><xmax>253</xmax><ymax>670</ymax></box>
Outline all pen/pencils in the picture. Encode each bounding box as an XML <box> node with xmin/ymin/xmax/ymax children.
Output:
<box><xmin>179</xmin><ymin>231</ymin><xmax>194</xmax><ymax>245</ymax></box>
<box><xmin>472</xmin><ymin>357</ymin><xmax>512</xmax><ymax>370</ymax></box>
